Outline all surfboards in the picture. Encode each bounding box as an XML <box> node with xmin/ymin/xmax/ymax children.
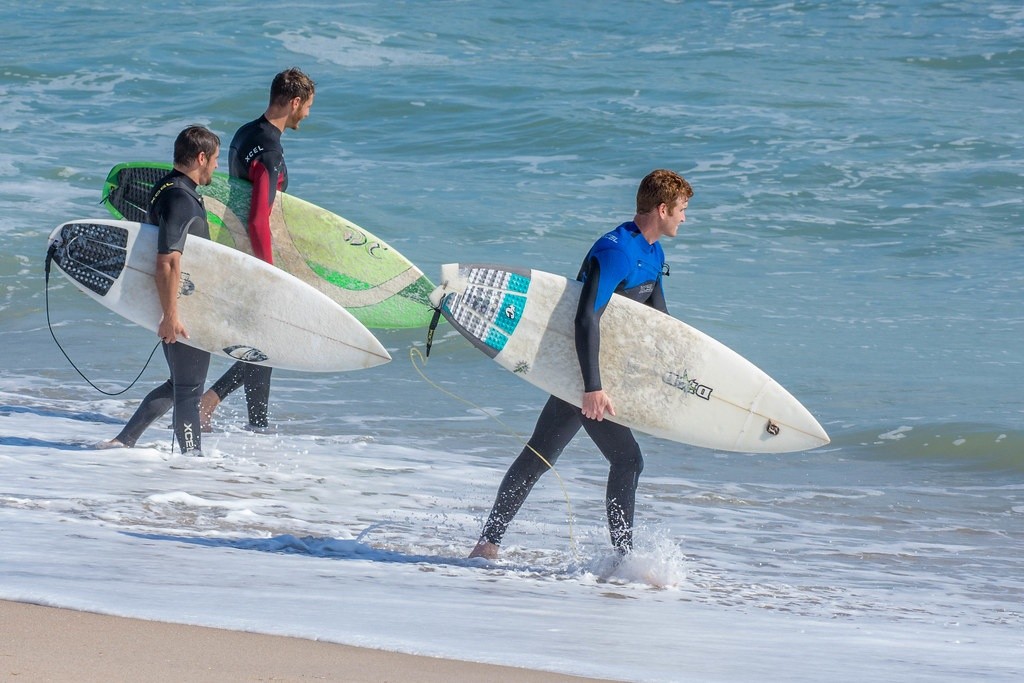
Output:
<box><xmin>47</xmin><ymin>218</ymin><xmax>392</xmax><ymax>373</ymax></box>
<box><xmin>440</xmin><ymin>260</ymin><xmax>832</xmax><ymax>454</ymax></box>
<box><xmin>103</xmin><ymin>160</ymin><xmax>449</xmax><ymax>330</ymax></box>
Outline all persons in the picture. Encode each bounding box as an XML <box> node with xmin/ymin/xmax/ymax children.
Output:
<box><xmin>469</xmin><ymin>168</ymin><xmax>694</xmax><ymax>560</ymax></box>
<box><xmin>199</xmin><ymin>68</ymin><xmax>315</xmax><ymax>429</ymax></box>
<box><xmin>112</xmin><ymin>124</ymin><xmax>221</xmax><ymax>457</ymax></box>
